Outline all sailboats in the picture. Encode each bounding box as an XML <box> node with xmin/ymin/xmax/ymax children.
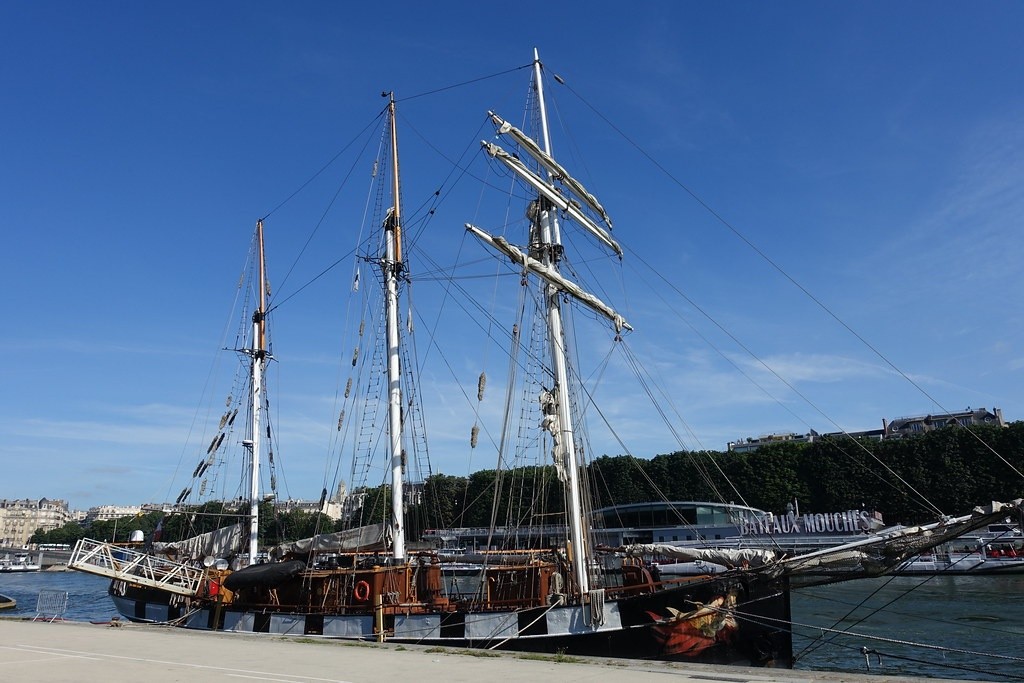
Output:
<box><xmin>67</xmin><ymin>48</ymin><xmax>1024</xmax><ymax>669</ymax></box>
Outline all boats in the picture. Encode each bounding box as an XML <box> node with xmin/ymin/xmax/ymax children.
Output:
<box><xmin>0</xmin><ymin>550</ymin><xmax>46</xmax><ymax>571</ymax></box>
<box><xmin>616</xmin><ymin>535</ymin><xmax>1024</xmax><ymax>575</ymax></box>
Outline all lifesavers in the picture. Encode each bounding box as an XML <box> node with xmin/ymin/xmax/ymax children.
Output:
<box><xmin>355</xmin><ymin>580</ymin><xmax>370</xmax><ymax>602</ymax></box>
